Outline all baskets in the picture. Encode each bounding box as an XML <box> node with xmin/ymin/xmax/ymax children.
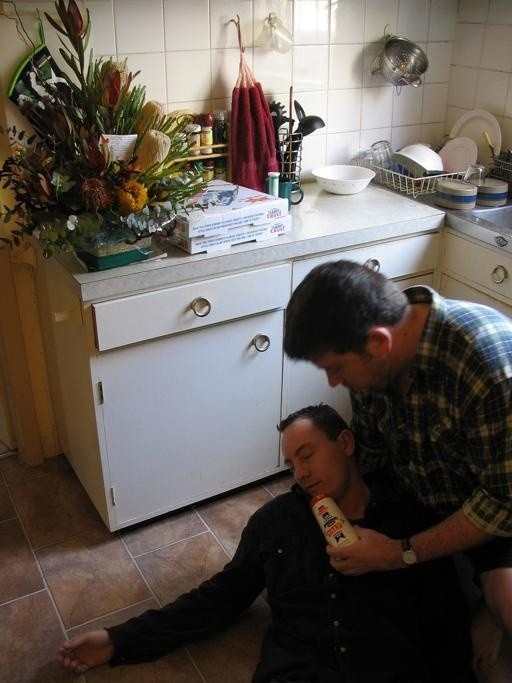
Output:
<box><xmin>490</xmin><ymin>155</ymin><xmax>512</xmax><ymax>185</ymax></box>
<box><xmin>362</xmin><ymin>158</ymin><xmax>486</xmax><ymax>199</ymax></box>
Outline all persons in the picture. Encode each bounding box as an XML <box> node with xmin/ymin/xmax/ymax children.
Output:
<box><xmin>283</xmin><ymin>258</ymin><xmax>512</xmax><ymax>673</ymax></box>
<box><xmin>59</xmin><ymin>403</ymin><xmax>477</xmax><ymax>683</ymax></box>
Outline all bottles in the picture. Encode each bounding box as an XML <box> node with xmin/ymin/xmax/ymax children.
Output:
<box><xmin>186</xmin><ymin>110</ymin><xmax>227</xmax><ymax>155</ymax></box>
<box><xmin>310</xmin><ymin>492</ymin><xmax>359</xmax><ymax>549</ymax></box>
<box><xmin>182</xmin><ymin>158</ymin><xmax>228</xmax><ymax>182</ymax></box>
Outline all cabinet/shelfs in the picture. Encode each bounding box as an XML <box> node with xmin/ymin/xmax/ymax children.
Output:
<box><xmin>76</xmin><ymin>229</ymin><xmax>440</xmax><ymax>535</ymax></box>
<box><xmin>440</xmin><ymin>227</ymin><xmax>509</xmax><ymax>319</ymax></box>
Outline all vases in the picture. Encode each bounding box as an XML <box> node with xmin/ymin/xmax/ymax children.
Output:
<box><xmin>71</xmin><ymin>220</ymin><xmax>157</xmax><ymax>269</ymax></box>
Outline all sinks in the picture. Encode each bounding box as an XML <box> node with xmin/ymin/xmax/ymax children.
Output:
<box><xmin>465</xmin><ymin>204</ymin><xmax>512</xmax><ymax>233</ymax></box>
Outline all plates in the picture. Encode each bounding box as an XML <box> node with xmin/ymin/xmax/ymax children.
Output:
<box><xmin>438</xmin><ymin>110</ymin><xmax>502</xmax><ymax>177</ymax></box>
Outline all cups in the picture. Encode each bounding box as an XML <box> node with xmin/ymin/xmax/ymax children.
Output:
<box><xmin>266</xmin><ymin>177</ymin><xmax>304</xmax><ymax>210</ymax></box>
<box><xmin>372</xmin><ymin>140</ymin><xmax>394</xmax><ymax>170</ymax></box>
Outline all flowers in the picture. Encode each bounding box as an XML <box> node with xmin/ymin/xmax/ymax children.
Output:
<box><xmin>0</xmin><ymin>0</ymin><xmax>218</xmax><ymax>259</ymax></box>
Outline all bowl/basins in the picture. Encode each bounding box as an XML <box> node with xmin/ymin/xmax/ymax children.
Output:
<box><xmin>393</xmin><ymin>142</ymin><xmax>443</xmax><ymax>177</ymax></box>
<box><xmin>436</xmin><ymin>178</ymin><xmax>509</xmax><ymax>209</ymax></box>
<box><xmin>311</xmin><ymin>165</ymin><xmax>376</xmax><ymax>195</ymax></box>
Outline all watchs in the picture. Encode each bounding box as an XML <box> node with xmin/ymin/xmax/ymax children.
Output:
<box><xmin>401</xmin><ymin>535</ymin><xmax>420</xmax><ymax>566</ymax></box>
